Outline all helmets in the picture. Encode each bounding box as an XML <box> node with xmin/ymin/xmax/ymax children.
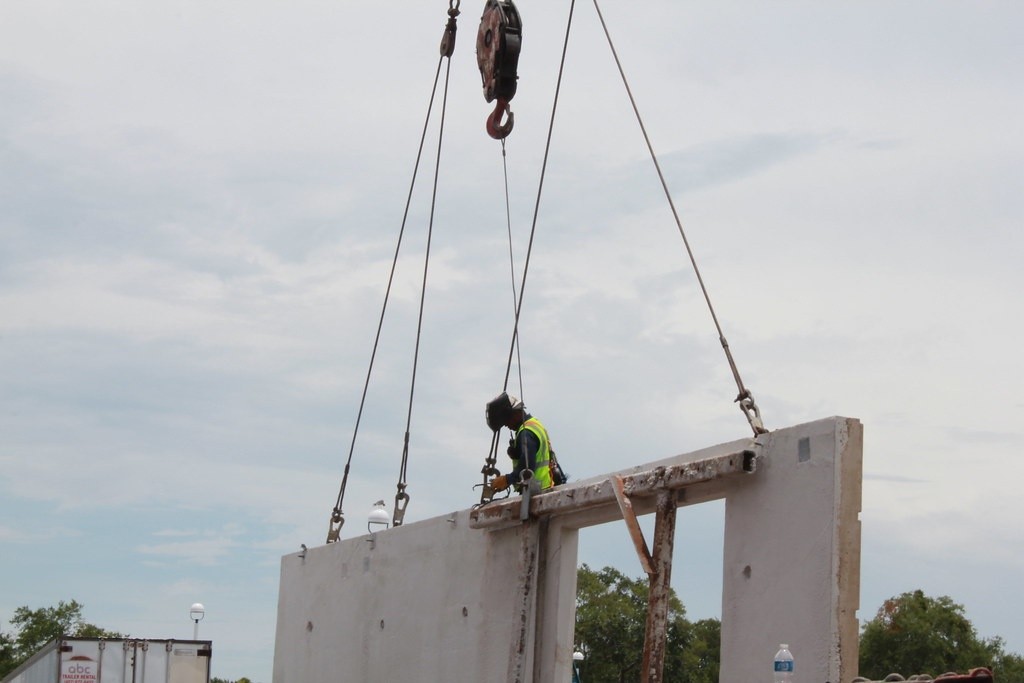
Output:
<box><xmin>507</xmin><ymin>394</ymin><xmax>523</xmax><ymax>412</ymax></box>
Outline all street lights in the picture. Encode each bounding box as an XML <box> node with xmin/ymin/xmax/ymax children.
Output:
<box><xmin>189</xmin><ymin>603</ymin><xmax>205</xmax><ymax>640</ymax></box>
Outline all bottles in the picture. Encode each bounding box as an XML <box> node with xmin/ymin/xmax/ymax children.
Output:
<box><xmin>773</xmin><ymin>643</ymin><xmax>793</xmax><ymax>683</ymax></box>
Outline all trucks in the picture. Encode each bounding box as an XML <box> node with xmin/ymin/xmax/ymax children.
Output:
<box><xmin>2</xmin><ymin>638</ymin><xmax>211</xmax><ymax>683</ymax></box>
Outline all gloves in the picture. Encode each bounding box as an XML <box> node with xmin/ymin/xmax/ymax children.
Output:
<box><xmin>491</xmin><ymin>476</ymin><xmax>508</xmax><ymax>492</ymax></box>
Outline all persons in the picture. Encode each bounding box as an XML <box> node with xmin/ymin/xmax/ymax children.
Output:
<box><xmin>486</xmin><ymin>392</ymin><xmax>554</xmax><ymax>495</ymax></box>
